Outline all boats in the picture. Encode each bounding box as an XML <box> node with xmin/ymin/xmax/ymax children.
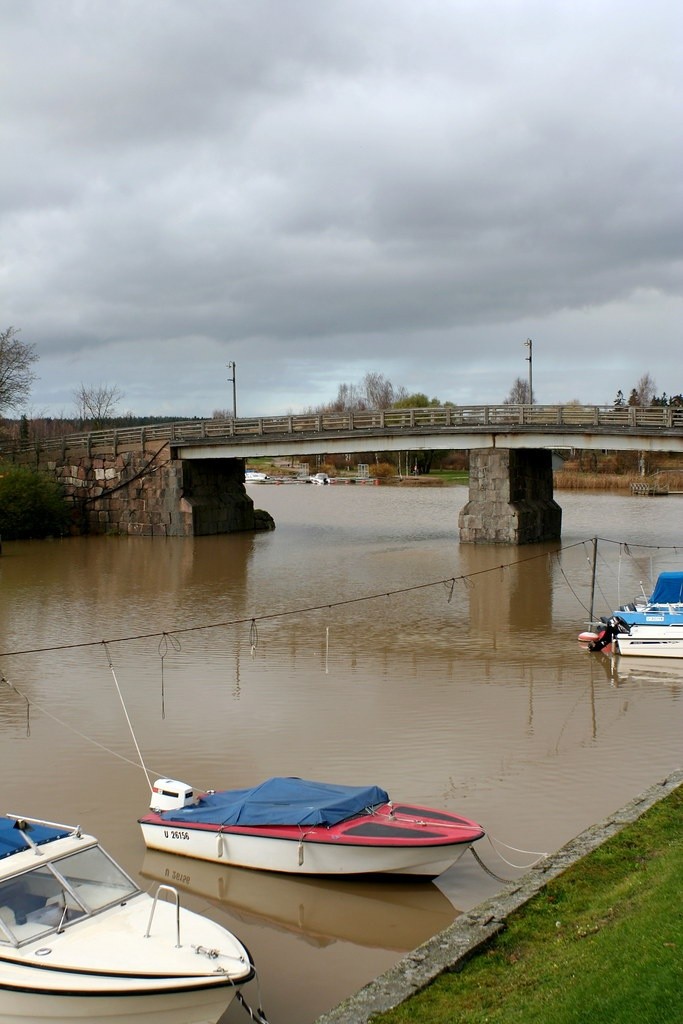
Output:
<box><xmin>137</xmin><ymin>777</ymin><xmax>485</xmax><ymax>876</ymax></box>
<box><xmin>245</xmin><ymin>468</ymin><xmax>270</xmax><ymax>482</ymax></box>
<box><xmin>577</xmin><ymin>595</ymin><xmax>683</xmax><ymax>658</ymax></box>
<box><xmin>0</xmin><ymin>814</ymin><xmax>256</xmax><ymax>1024</ymax></box>
<box><xmin>310</xmin><ymin>472</ymin><xmax>330</xmax><ymax>485</ymax></box>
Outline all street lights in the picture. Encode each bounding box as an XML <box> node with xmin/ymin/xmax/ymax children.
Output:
<box><xmin>226</xmin><ymin>360</ymin><xmax>236</xmax><ymax>419</ymax></box>
<box><xmin>524</xmin><ymin>337</ymin><xmax>533</xmax><ymax>403</ymax></box>
